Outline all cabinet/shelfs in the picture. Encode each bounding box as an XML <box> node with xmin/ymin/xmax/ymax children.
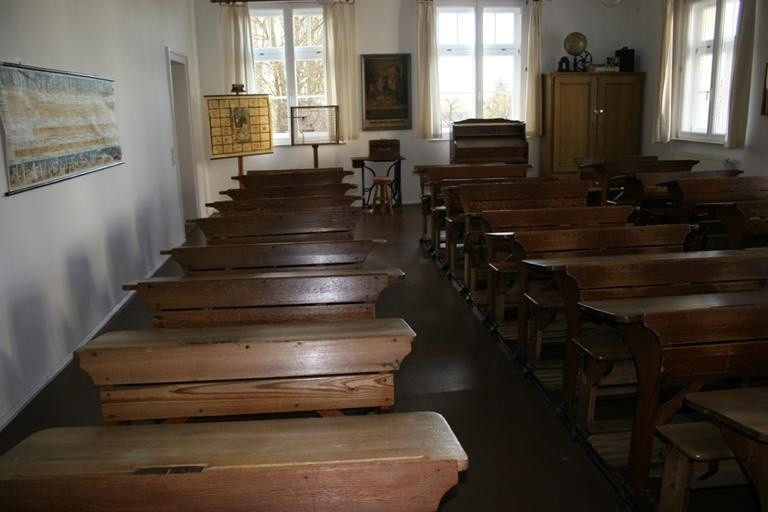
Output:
<box><xmin>543</xmin><ymin>70</ymin><xmax>643</xmax><ymax>183</ymax></box>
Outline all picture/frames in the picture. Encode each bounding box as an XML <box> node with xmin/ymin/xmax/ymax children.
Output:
<box><xmin>361</xmin><ymin>52</ymin><xmax>411</xmax><ymax>131</ymax></box>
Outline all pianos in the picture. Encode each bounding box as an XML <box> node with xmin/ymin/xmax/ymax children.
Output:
<box><xmin>449</xmin><ymin>118</ymin><xmax>528</xmax><ymax>165</ymax></box>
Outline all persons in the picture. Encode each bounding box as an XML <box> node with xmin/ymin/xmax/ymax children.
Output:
<box><xmin>382</xmin><ymin>64</ymin><xmax>406</xmax><ymax>101</ymax></box>
<box><xmin>369</xmin><ymin>75</ymin><xmax>388</xmax><ymax>95</ymax></box>
